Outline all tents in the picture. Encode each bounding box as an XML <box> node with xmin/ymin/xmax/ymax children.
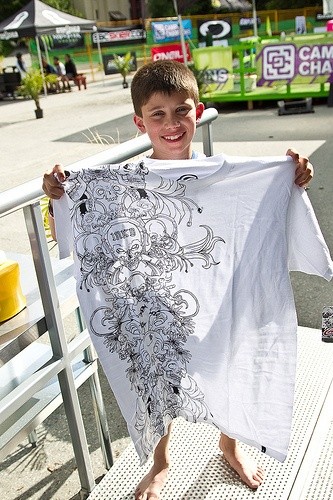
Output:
<box><xmin>0</xmin><ymin>0</ymin><xmax>106</xmax><ymax>96</ymax></box>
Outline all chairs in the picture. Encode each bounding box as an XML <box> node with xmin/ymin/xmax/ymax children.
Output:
<box><xmin>0</xmin><ymin>66</ymin><xmax>23</xmax><ymax>100</ymax></box>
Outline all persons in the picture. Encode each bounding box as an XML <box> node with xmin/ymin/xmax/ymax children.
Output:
<box><xmin>40</xmin><ymin>57</ymin><xmax>315</xmax><ymax>500</ymax></box>
<box><xmin>16</xmin><ymin>51</ymin><xmax>77</xmax><ymax>92</ymax></box>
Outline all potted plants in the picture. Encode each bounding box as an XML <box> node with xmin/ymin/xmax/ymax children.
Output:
<box><xmin>17</xmin><ymin>67</ymin><xmax>56</xmax><ymax>118</ymax></box>
<box><xmin>113</xmin><ymin>52</ymin><xmax>133</xmax><ymax>88</ymax></box>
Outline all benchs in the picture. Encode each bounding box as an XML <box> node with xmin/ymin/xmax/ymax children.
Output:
<box><xmin>57</xmin><ymin>73</ymin><xmax>86</xmax><ymax>93</ymax></box>
